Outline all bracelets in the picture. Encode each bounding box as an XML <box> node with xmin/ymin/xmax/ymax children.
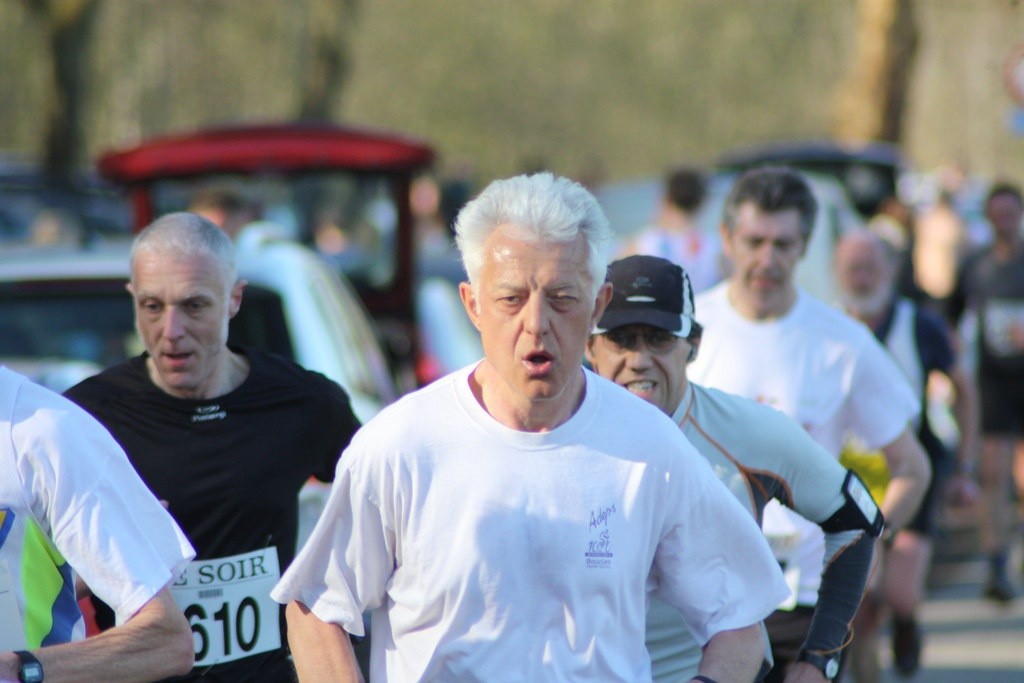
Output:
<box><xmin>690</xmin><ymin>674</ymin><xmax>715</xmax><ymax>683</ymax></box>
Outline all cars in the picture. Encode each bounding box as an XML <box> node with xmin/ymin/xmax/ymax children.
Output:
<box><xmin>0</xmin><ymin>128</ymin><xmax>900</xmax><ymax>423</ymax></box>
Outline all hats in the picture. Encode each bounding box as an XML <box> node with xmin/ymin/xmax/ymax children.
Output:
<box><xmin>589</xmin><ymin>256</ymin><xmax>696</xmax><ymax>338</ymax></box>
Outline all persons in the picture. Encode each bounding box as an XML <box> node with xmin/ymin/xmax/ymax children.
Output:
<box><xmin>585</xmin><ymin>252</ymin><xmax>887</xmax><ymax>683</ymax></box>
<box><xmin>263</xmin><ymin>172</ymin><xmax>789</xmax><ymax>681</ymax></box>
<box><xmin>50</xmin><ymin>209</ymin><xmax>364</xmax><ymax>683</ymax></box>
<box><xmin>1</xmin><ymin>356</ymin><xmax>201</xmax><ymax>683</ymax></box>
<box><xmin>187</xmin><ymin>164</ymin><xmax>1024</xmax><ymax>683</ymax></box>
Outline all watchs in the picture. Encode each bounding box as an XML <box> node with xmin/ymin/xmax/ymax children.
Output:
<box><xmin>13</xmin><ymin>649</ymin><xmax>44</xmax><ymax>683</ymax></box>
<box><xmin>793</xmin><ymin>650</ymin><xmax>839</xmax><ymax>680</ymax></box>
